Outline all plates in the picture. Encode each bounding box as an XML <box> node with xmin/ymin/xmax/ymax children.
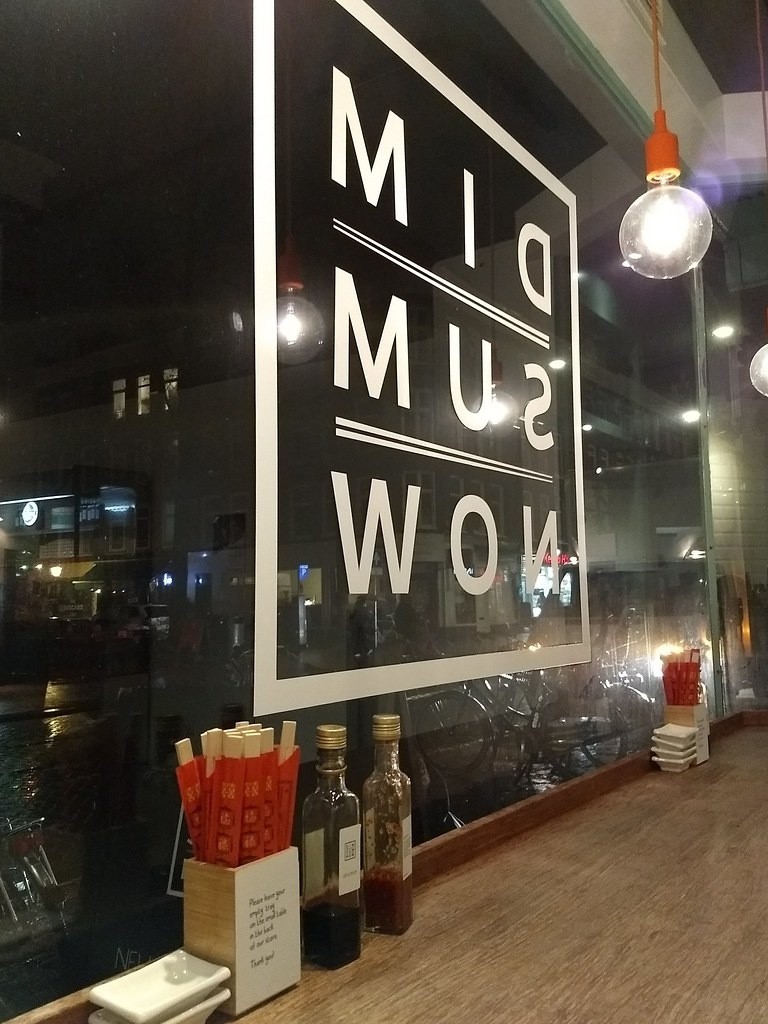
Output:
<box><xmin>651</xmin><ymin>723</ymin><xmax>699</xmax><ymax>773</ymax></box>
<box><xmin>88</xmin><ymin>950</ymin><xmax>232</xmax><ymax>1024</ymax></box>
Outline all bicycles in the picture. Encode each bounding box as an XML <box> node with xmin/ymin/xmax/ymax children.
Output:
<box><xmin>414</xmin><ymin>591</ymin><xmax>670</xmax><ymax>786</ymax></box>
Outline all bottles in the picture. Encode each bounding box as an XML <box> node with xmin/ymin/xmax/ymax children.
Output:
<box><xmin>361</xmin><ymin>714</ymin><xmax>415</xmax><ymax>937</ymax></box>
<box><xmin>299</xmin><ymin>724</ymin><xmax>362</xmax><ymax>970</ymax></box>
